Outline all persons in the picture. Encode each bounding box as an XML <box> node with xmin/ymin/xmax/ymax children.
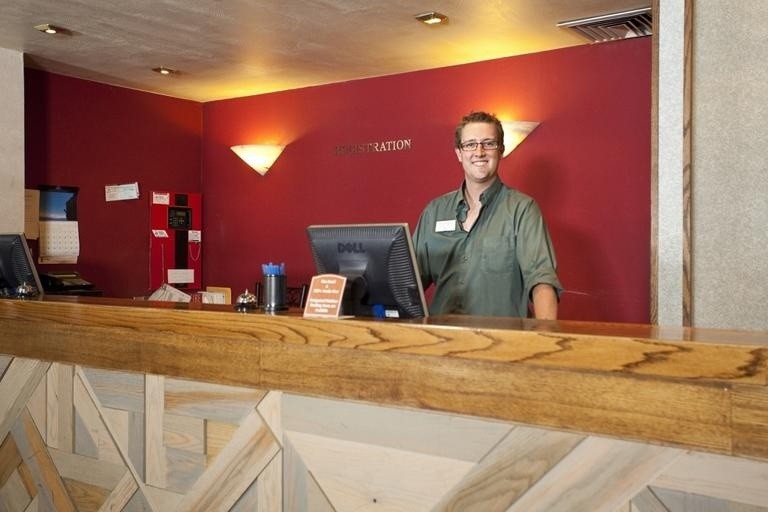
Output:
<box><xmin>411</xmin><ymin>111</ymin><xmax>564</xmax><ymax>319</ymax></box>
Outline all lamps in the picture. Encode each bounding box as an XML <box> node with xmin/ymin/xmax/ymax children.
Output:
<box><xmin>492</xmin><ymin>117</ymin><xmax>545</xmax><ymax>162</ymax></box>
<box><xmin>225</xmin><ymin>142</ymin><xmax>286</xmax><ymax>177</ymax></box>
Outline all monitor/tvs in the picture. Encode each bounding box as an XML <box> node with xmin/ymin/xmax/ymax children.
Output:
<box><xmin>306</xmin><ymin>224</ymin><xmax>428</xmax><ymax>317</ymax></box>
<box><xmin>0</xmin><ymin>233</ymin><xmax>44</xmax><ymax>295</ymax></box>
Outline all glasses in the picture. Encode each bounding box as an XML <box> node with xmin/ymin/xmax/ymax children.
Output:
<box><xmin>457</xmin><ymin>139</ymin><xmax>501</xmax><ymax>153</ymax></box>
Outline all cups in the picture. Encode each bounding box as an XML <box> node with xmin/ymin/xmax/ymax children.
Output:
<box><xmin>263</xmin><ymin>273</ymin><xmax>288</xmax><ymax>313</ymax></box>
